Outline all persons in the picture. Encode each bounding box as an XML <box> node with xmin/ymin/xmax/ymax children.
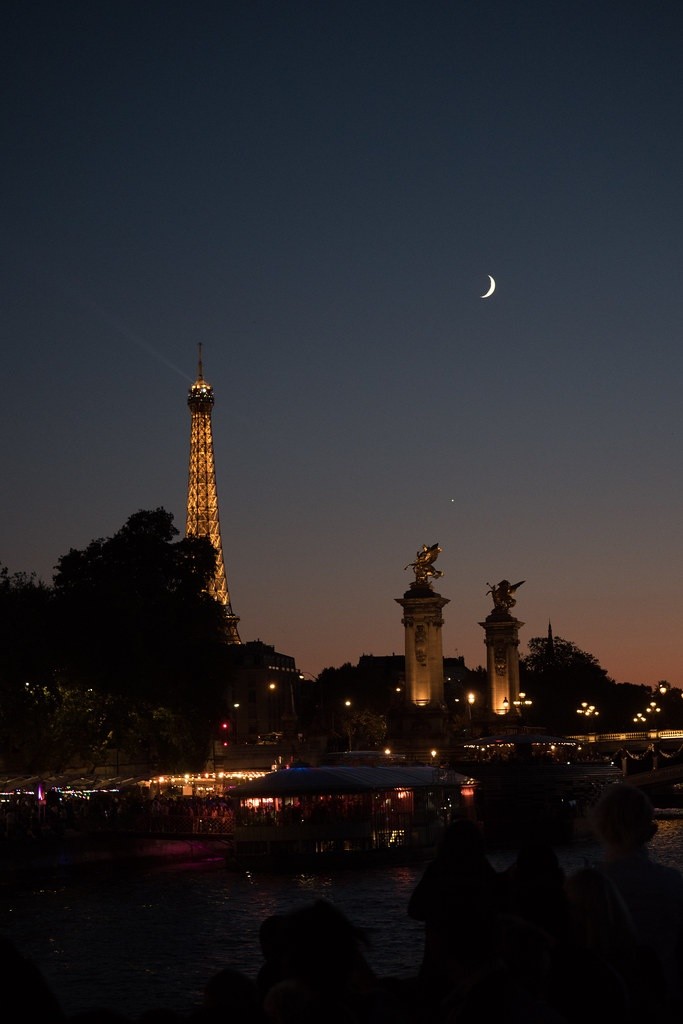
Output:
<box><xmin>0</xmin><ymin>784</ymin><xmax>683</xmax><ymax>1024</ymax></box>
<box><xmin>414</xmin><ymin>625</ymin><xmax>427</xmax><ymax>662</ymax></box>
